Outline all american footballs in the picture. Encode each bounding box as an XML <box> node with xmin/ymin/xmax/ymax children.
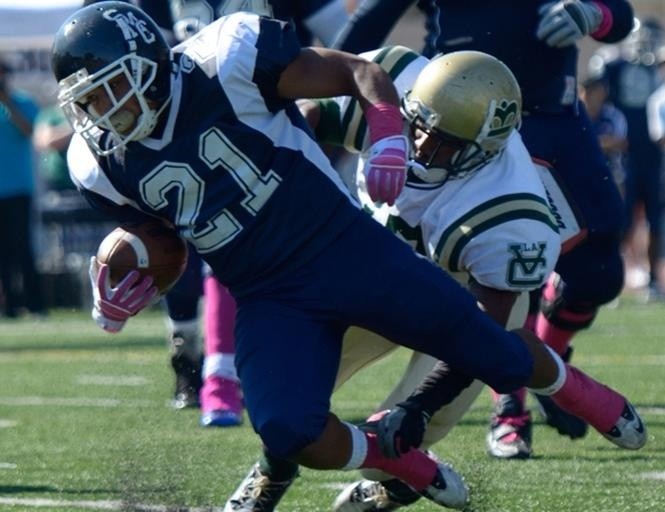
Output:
<box><xmin>97</xmin><ymin>225</ymin><xmax>187</xmax><ymax>295</ymax></box>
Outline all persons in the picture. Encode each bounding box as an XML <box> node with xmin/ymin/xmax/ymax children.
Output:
<box><xmin>221</xmin><ymin>39</ymin><xmax>590</xmax><ymax>511</ymax></box>
<box><xmin>47</xmin><ymin>0</ymin><xmax>647</xmax><ymax>510</ymax></box>
<box><xmin>320</xmin><ymin>0</ymin><xmax>638</xmax><ymax>464</ymax></box>
<box><xmin>84</xmin><ymin>0</ymin><xmax>315</xmax><ymax>430</ymax></box>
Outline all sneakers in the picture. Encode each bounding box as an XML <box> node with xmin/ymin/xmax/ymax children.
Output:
<box><xmin>202</xmin><ymin>409</ymin><xmax>243</xmax><ymax>426</ymax></box>
<box><xmin>364</xmin><ymin>409</ymin><xmax>468</xmax><ymax>509</ymax></box>
<box><xmin>534</xmin><ymin>351</ymin><xmax>588</xmax><ymax>440</ymax></box>
<box><xmin>223</xmin><ymin>461</ymin><xmax>297</xmax><ymax>512</ymax></box>
<box><xmin>560</xmin><ymin>361</ymin><xmax>649</xmax><ymax>450</ymax></box>
<box><xmin>332</xmin><ymin>450</ymin><xmax>439</xmax><ymax>511</ymax></box>
<box><xmin>168</xmin><ymin>355</ymin><xmax>202</xmax><ymax>410</ymax></box>
<box><xmin>488</xmin><ymin>412</ymin><xmax>534</xmax><ymax>460</ymax></box>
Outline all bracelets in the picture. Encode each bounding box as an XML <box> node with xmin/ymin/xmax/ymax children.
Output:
<box><xmin>363</xmin><ymin>103</ymin><xmax>406</xmax><ymax>143</ymax></box>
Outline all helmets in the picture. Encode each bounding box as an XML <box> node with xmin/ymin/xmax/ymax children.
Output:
<box><xmin>400</xmin><ymin>51</ymin><xmax>524</xmax><ymax>188</ymax></box>
<box><xmin>52</xmin><ymin>2</ymin><xmax>171</xmax><ymax>114</ymax></box>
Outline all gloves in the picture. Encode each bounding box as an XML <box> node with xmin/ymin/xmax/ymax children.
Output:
<box><xmin>535</xmin><ymin>1</ymin><xmax>613</xmax><ymax>51</ymax></box>
<box><xmin>357</xmin><ymin>403</ymin><xmax>432</xmax><ymax>458</ymax></box>
<box><xmin>86</xmin><ymin>257</ymin><xmax>157</xmax><ymax>336</ymax></box>
<box><xmin>363</xmin><ymin>102</ymin><xmax>410</xmax><ymax>207</ymax></box>
